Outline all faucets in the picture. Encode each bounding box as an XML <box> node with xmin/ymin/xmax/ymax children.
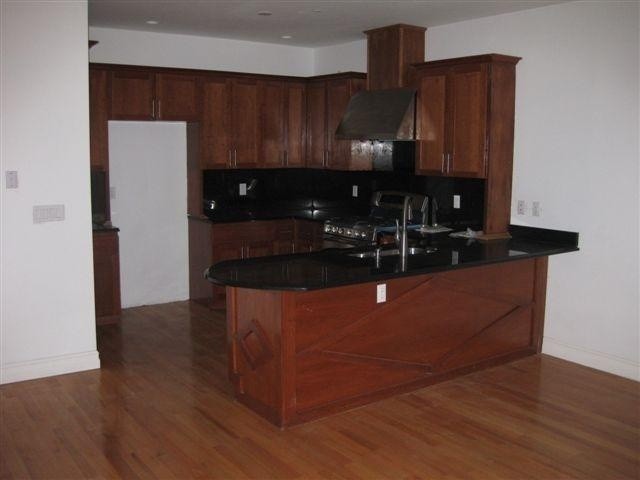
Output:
<box><xmin>420</xmin><ymin>196</ymin><xmax>429</xmax><ymax>224</ymax></box>
<box><xmin>432</xmin><ymin>198</ymin><xmax>438</xmax><ymax>226</ymax></box>
<box><xmin>403</xmin><ymin>196</ymin><xmax>413</xmax><ymax>231</ymax></box>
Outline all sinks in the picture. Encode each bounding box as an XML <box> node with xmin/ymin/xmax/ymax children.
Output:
<box><xmin>344</xmin><ymin>247</ymin><xmax>429</xmax><ymax>259</ymax></box>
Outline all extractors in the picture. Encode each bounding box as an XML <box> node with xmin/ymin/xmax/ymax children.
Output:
<box><xmin>334</xmin><ymin>88</ymin><xmax>417</xmax><ymax>143</ymax></box>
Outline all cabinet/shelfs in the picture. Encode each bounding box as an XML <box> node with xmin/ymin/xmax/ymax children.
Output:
<box><xmin>187</xmin><ymin>74</ymin><xmax>373</xmax><ymax>171</ymax></box>
<box><xmin>94</xmin><ymin>233</ymin><xmax>122</xmax><ymax>317</ymax></box>
<box><xmin>295</xmin><ymin>220</ymin><xmax>323</xmax><ymax>253</ymax></box>
<box><xmin>276</xmin><ymin>218</ymin><xmax>295</xmax><ymax>254</ymax></box>
<box><xmin>190</xmin><ymin>218</ymin><xmax>276</xmax><ymax>309</ymax></box>
<box><xmin>410</xmin><ymin>54</ymin><xmax>521</xmax><ymax>179</ymax></box>
<box><xmin>108</xmin><ymin>66</ymin><xmax>196</xmax><ymax>119</ymax></box>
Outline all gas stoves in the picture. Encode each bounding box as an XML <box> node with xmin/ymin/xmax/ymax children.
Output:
<box><xmin>329</xmin><ymin>214</ymin><xmax>411</xmax><ymax>229</ymax></box>
